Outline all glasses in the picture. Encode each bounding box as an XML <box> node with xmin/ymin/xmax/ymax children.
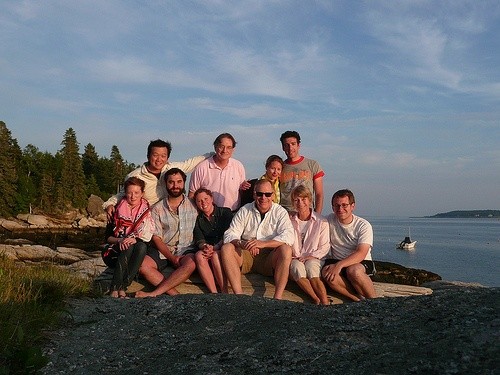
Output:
<box><xmin>333</xmin><ymin>202</ymin><xmax>354</xmax><ymax>208</ymax></box>
<box><xmin>254</xmin><ymin>191</ymin><xmax>273</xmax><ymax>197</ymax></box>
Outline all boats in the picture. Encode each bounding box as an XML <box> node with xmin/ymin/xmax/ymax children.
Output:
<box><xmin>395</xmin><ymin>236</ymin><xmax>417</xmax><ymax>249</ymax></box>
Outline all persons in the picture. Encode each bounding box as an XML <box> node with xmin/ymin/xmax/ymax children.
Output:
<box><xmin>101</xmin><ymin>177</ymin><xmax>154</xmax><ymax>298</ymax></box>
<box><xmin>240</xmin><ymin>130</ymin><xmax>324</xmax><ymax>214</ymax></box>
<box><xmin>241</xmin><ymin>154</ymin><xmax>283</xmax><ymax>207</ymax></box>
<box><xmin>189</xmin><ymin>133</ymin><xmax>246</xmax><ymax>216</ymax></box>
<box><xmin>221</xmin><ymin>179</ymin><xmax>295</xmax><ymax>300</ymax></box>
<box><xmin>101</xmin><ymin>139</ymin><xmax>216</xmax><ymax>221</ymax></box>
<box><xmin>134</xmin><ymin>168</ymin><xmax>199</xmax><ymax>299</ymax></box>
<box><xmin>321</xmin><ymin>189</ymin><xmax>376</xmax><ymax>301</ymax></box>
<box><xmin>193</xmin><ymin>188</ymin><xmax>234</xmax><ymax>294</ymax></box>
<box><xmin>288</xmin><ymin>185</ymin><xmax>331</xmax><ymax>305</ymax></box>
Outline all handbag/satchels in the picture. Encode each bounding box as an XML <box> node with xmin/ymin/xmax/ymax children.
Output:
<box><xmin>101</xmin><ymin>204</ymin><xmax>150</xmax><ymax>268</ymax></box>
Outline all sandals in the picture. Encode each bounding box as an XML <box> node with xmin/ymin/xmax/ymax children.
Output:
<box><xmin>110</xmin><ymin>291</ymin><xmax>131</xmax><ymax>300</ymax></box>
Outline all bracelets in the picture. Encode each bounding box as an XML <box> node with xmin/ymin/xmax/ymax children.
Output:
<box><xmin>237</xmin><ymin>240</ymin><xmax>244</xmax><ymax>247</ymax></box>
